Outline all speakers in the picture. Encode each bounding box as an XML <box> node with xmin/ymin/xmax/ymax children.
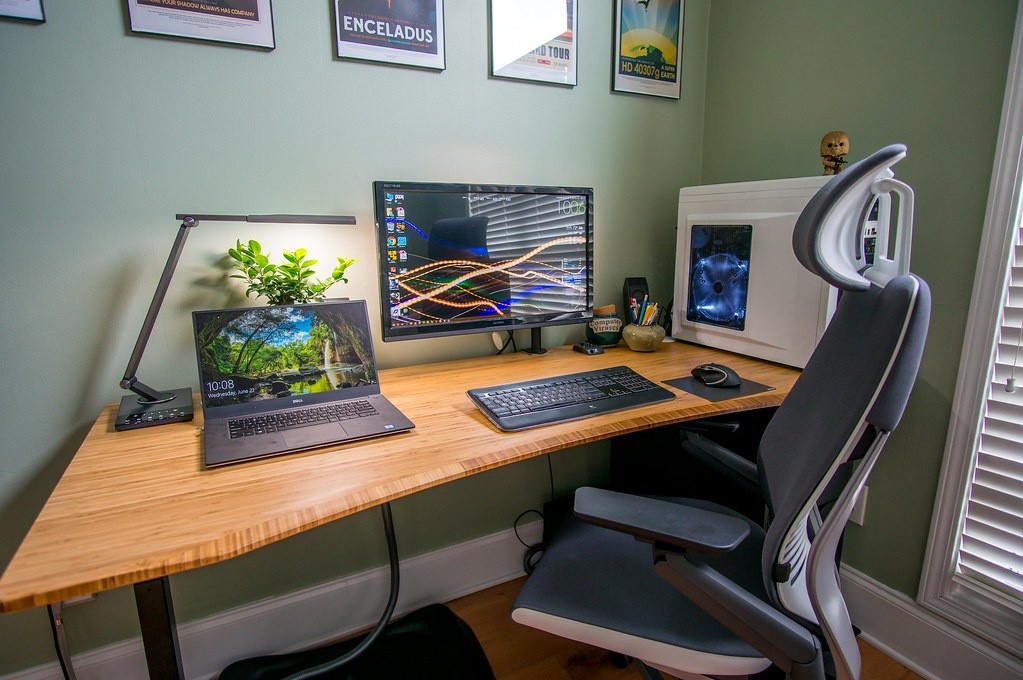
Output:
<box><xmin>623</xmin><ymin>275</ymin><xmax>649</xmax><ymax>326</ymax></box>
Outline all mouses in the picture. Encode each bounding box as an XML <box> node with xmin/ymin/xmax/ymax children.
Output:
<box><xmin>690</xmin><ymin>362</ymin><xmax>743</xmax><ymax>388</ymax></box>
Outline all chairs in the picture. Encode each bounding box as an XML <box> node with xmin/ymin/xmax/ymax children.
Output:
<box><xmin>508</xmin><ymin>144</ymin><xmax>933</xmax><ymax>680</ymax></box>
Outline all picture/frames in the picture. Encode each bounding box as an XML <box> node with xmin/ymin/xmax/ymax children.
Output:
<box><xmin>125</xmin><ymin>0</ymin><xmax>277</xmax><ymax>50</ymax></box>
<box><xmin>485</xmin><ymin>0</ymin><xmax>578</xmax><ymax>87</ymax></box>
<box><xmin>333</xmin><ymin>0</ymin><xmax>447</xmax><ymax>72</ymax></box>
<box><xmin>611</xmin><ymin>1</ymin><xmax>685</xmax><ymax>101</ymax></box>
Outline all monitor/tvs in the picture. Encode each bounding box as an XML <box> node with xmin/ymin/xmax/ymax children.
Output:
<box><xmin>372</xmin><ymin>181</ymin><xmax>595</xmax><ymax>355</ymax></box>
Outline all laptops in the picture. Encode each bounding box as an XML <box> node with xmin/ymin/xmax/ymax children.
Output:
<box><xmin>191</xmin><ymin>299</ymin><xmax>415</xmax><ymax>468</ymax></box>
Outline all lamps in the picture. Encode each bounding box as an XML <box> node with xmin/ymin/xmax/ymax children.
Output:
<box><xmin>111</xmin><ymin>213</ymin><xmax>358</xmax><ymax>432</ymax></box>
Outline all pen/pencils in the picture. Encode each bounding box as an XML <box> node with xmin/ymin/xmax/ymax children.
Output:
<box><xmin>629</xmin><ymin>295</ymin><xmax>660</xmax><ymax>325</ymax></box>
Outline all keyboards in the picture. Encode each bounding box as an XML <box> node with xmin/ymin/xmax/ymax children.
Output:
<box><xmin>465</xmin><ymin>365</ymin><xmax>677</xmax><ymax>433</ymax></box>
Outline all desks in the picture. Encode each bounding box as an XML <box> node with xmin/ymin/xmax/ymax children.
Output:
<box><xmin>0</xmin><ymin>325</ymin><xmax>808</xmax><ymax>680</ymax></box>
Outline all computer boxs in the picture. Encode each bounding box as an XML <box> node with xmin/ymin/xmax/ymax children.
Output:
<box><xmin>671</xmin><ymin>174</ymin><xmax>891</xmax><ymax>369</ymax></box>
